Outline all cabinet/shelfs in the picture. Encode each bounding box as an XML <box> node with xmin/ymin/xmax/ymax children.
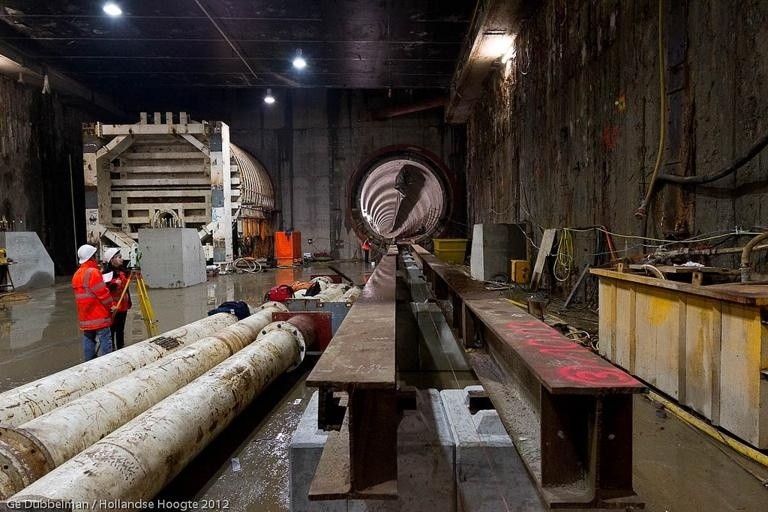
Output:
<box><xmin>588</xmin><ymin>264</ymin><xmax>768</xmax><ymax>451</ymax></box>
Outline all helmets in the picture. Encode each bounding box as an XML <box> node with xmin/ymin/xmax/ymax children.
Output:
<box><xmin>104</xmin><ymin>247</ymin><xmax>120</xmax><ymax>264</ymax></box>
<box><xmin>78</xmin><ymin>243</ymin><xmax>97</xmax><ymax>265</ymax></box>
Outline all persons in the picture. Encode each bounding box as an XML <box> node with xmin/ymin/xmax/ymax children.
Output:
<box><xmin>71</xmin><ymin>243</ymin><xmax>117</xmax><ymax>363</ymax></box>
<box><xmin>361</xmin><ymin>236</ymin><xmax>374</xmax><ymax>263</ymax></box>
<box><xmin>100</xmin><ymin>246</ymin><xmax>143</xmax><ymax>350</ymax></box>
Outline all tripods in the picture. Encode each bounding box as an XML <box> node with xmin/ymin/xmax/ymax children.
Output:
<box><xmin>96</xmin><ymin>269</ymin><xmax>159</xmax><ymax>338</ymax></box>
